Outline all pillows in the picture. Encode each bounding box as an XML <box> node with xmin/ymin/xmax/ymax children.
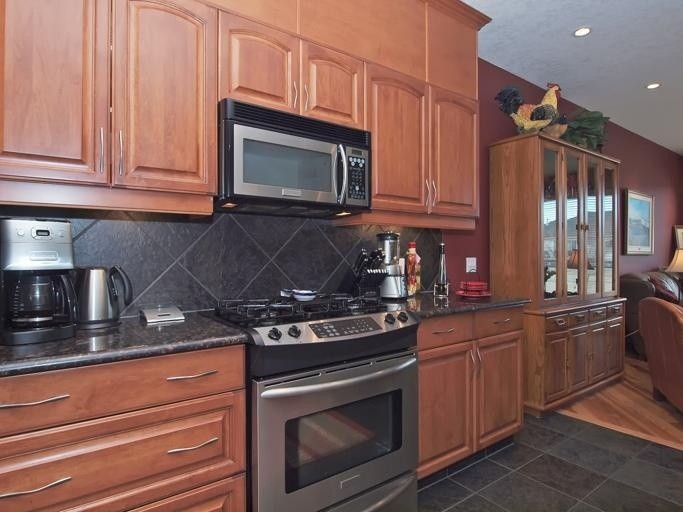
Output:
<box><xmin>647</xmin><ymin>272</ymin><xmax>679</xmax><ymax>301</ymax></box>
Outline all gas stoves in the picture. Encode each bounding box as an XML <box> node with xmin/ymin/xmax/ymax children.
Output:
<box><xmin>198</xmin><ymin>290</ymin><xmax>419</xmax><ymax>348</ymax></box>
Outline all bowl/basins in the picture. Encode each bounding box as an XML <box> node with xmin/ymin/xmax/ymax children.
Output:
<box><xmin>294</xmin><ymin>289</ymin><xmax>317</xmax><ymax>302</ymax></box>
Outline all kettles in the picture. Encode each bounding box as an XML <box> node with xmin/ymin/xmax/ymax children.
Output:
<box><xmin>77</xmin><ymin>265</ymin><xmax>133</xmax><ymax>330</ymax></box>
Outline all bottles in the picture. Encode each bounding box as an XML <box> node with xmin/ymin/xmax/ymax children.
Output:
<box><xmin>404</xmin><ymin>242</ymin><xmax>421</xmax><ymax>296</ymax></box>
<box><xmin>438</xmin><ymin>244</ymin><xmax>447</xmax><ymax>284</ymax></box>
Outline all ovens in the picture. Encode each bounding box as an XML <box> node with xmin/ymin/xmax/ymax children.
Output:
<box><xmin>248</xmin><ymin>348</ymin><xmax>419</xmax><ymax>512</ymax></box>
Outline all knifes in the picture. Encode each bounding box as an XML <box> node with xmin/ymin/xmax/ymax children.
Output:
<box><xmin>354</xmin><ymin>247</ymin><xmax>385</xmax><ymax>273</ymax></box>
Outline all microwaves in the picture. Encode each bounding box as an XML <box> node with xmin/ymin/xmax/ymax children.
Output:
<box><xmin>215</xmin><ymin>98</ymin><xmax>372</xmax><ymax>220</ymax></box>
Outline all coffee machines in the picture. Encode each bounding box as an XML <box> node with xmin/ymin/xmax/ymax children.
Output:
<box><xmin>0</xmin><ymin>216</ymin><xmax>77</xmax><ymax>345</ymax></box>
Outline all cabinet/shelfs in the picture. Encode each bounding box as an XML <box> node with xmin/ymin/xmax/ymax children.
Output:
<box><xmin>417</xmin><ymin>306</ymin><xmax>527</xmax><ymax>479</ymax></box>
<box><xmin>587</xmin><ymin>302</ymin><xmax>625</xmax><ymax>384</ymax></box>
<box><xmin>363</xmin><ymin>60</ymin><xmax>480</xmax><ymax>218</ymax></box>
<box><xmin>538</xmin><ymin>140</ymin><xmax>587</xmax><ymax>306</ymax></box>
<box><xmin>0</xmin><ymin>341</ymin><xmax>250</xmax><ymax>512</ymax></box>
<box><xmin>544</xmin><ymin>309</ymin><xmax>588</xmax><ymax>402</ymax></box>
<box><xmin>1</xmin><ymin>0</ymin><xmax>218</xmax><ymax>196</ymax></box>
<box><xmin>217</xmin><ymin>10</ymin><xmax>366</xmax><ymax>129</ymax></box>
<box><xmin>587</xmin><ymin>154</ymin><xmax>619</xmax><ymax>299</ymax></box>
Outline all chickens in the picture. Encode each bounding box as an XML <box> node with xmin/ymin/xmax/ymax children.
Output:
<box><xmin>494</xmin><ymin>82</ymin><xmax>562</xmax><ymax>135</ymax></box>
<box><xmin>541</xmin><ymin>114</ymin><xmax>569</xmax><ymax>139</ymax></box>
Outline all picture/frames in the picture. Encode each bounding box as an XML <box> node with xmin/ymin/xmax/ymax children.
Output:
<box><xmin>623</xmin><ymin>188</ymin><xmax>655</xmax><ymax>255</ymax></box>
<box><xmin>673</xmin><ymin>225</ymin><xmax>683</xmax><ymax>250</ymax></box>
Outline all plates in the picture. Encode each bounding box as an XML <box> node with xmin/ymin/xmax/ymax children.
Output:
<box><xmin>454</xmin><ymin>282</ymin><xmax>493</xmax><ymax>301</ymax></box>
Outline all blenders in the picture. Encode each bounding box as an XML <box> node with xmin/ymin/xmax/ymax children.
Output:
<box><xmin>376</xmin><ymin>232</ymin><xmax>407</xmax><ymax>311</ymax></box>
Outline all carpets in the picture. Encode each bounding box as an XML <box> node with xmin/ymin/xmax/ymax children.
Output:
<box><xmin>553</xmin><ymin>344</ymin><xmax>683</xmax><ymax>450</ymax></box>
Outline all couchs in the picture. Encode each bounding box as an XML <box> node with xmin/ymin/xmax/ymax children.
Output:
<box><xmin>619</xmin><ymin>268</ymin><xmax>683</xmax><ymax>360</ymax></box>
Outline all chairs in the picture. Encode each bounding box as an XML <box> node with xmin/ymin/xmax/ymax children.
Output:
<box><xmin>638</xmin><ymin>296</ymin><xmax>683</xmax><ymax>414</ymax></box>
